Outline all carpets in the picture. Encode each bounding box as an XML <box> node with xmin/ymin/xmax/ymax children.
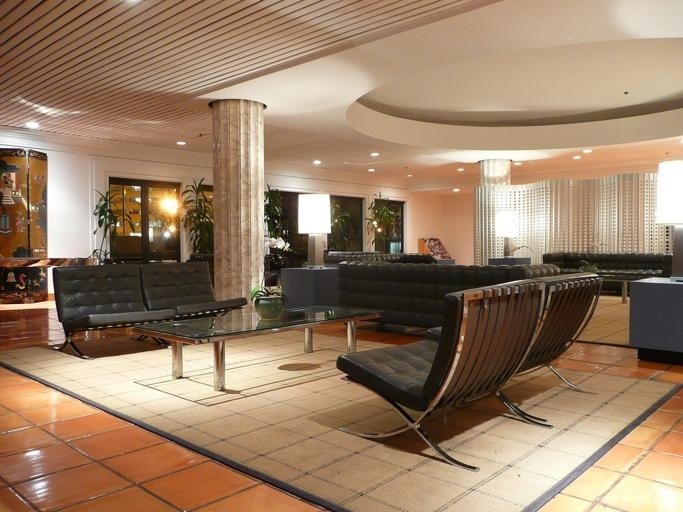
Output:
<box><xmin>570</xmin><ymin>294</ymin><xmax>630</xmax><ymax>346</ymax></box>
<box><xmin>0</xmin><ymin>334</ymin><xmax>683</xmax><ymax>512</ymax></box>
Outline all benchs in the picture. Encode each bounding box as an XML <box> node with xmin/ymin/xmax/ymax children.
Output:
<box><xmin>132</xmin><ymin>261</ymin><xmax>248</xmax><ymax>350</ymax></box>
<box><xmin>412</xmin><ymin>270</ymin><xmax>609</xmax><ymax>430</ymax></box>
<box><xmin>280</xmin><ymin>248</ymin><xmax>630</xmax><ymax>317</ymax></box>
<box><xmin>52</xmin><ymin>262</ymin><xmax>178</xmax><ymax>361</ymax></box>
<box><xmin>335</xmin><ymin>277</ymin><xmax>551</xmax><ymax>470</ymax></box>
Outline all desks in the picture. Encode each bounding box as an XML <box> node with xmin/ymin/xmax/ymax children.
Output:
<box><xmin>132</xmin><ymin>303</ymin><xmax>385</xmax><ymax>392</ymax></box>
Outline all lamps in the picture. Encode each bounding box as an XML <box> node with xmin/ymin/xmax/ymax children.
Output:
<box><xmin>497</xmin><ymin>210</ymin><xmax>519</xmax><ymax>262</ymax></box>
<box><xmin>480</xmin><ymin>159</ymin><xmax>511</xmax><ymax>188</ymax></box>
<box><xmin>655</xmin><ymin>160</ymin><xmax>683</xmax><ymax>282</ymax></box>
<box><xmin>297</xmin><ymin>194</ymin><xmax>332</xmax><ymax>267</ymax></box>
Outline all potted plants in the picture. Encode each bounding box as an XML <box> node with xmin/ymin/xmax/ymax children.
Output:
<box><xmin>251</xmin><ymin>283</ymin><xmax>288</xmax><ymax>320</ymax></box>
<box><xmin>180</xmin><ymin>176</ymin><xmax>298</xmax><ymax>322</ymax></box>
